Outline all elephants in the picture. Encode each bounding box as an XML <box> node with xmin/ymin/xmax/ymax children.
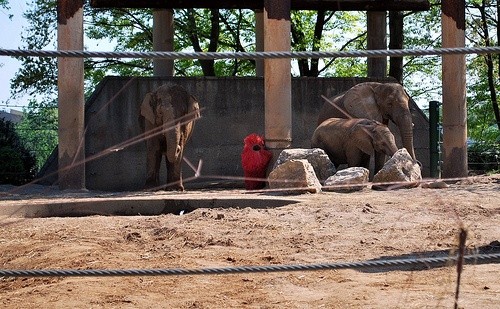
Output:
<box><xmin>140</xmin><ymin>82</ymin><xmax>200</xmax><ymax>191</ymax></box>
<box><xmin>311</xmin><ymin>118</ymin><xmax>398</xmax><ymax>168</ymax></box>
<box><xmin>317</xmin><ymin>81</ymin><xmax>416</xmax><ymax>174</ymax></box>
